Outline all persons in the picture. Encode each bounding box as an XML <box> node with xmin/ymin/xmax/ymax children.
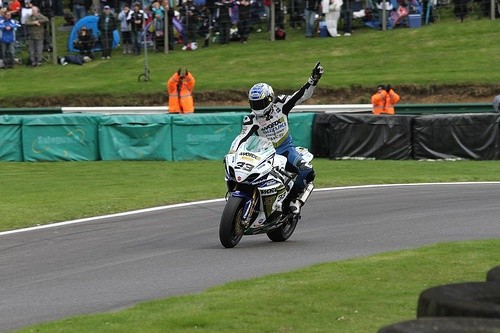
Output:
<box><xmin>287</xmin><ymin>0</ymin><xmax>434</xmax><ymax>38</ymax></box>
<box><xmin>223</xmin><ymin>61</ymin><xmax>325</xmax><ymax>214</ymax></box>
<box><xmin>170</xmin><ymin>0</ymin><xmax>285</xmax><ymax>50</ymax></box>
<box><xmin>371</xmin><ymin>83</ymin><xmax>400</xmax><ymax>115</ymax></box>
<box><xmin>48</xmin><ymin>0</ymin><xmax>174</xmax><ymax>65</ymax></box>
<box><xmin>167</xmin><ymin>67</ymin><xmax>195</xmax><ymax>113</ymax></box>
<box><xmin>0</xmin><ymin>0</ymin><xmax>53</xmax><ymax>68</ymax></box>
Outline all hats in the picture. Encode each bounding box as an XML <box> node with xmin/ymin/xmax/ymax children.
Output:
<box><xmin>104</xmin><ymin>6</ymin><xmax>110</xmax><ymax>9</ymax></box>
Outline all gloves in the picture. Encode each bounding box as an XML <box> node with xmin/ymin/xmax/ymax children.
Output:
<box><xmin>385</xmin><ymin>84</ymin><xmax>391</xmax><ymax>92</ymax></box>
<box><xmin>312</xmin><ymin>61</ymin><xmax>323</xmax><ymax>81</ymax></box>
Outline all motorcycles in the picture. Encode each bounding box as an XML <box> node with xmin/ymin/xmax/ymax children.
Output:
<box><xmin>218</xmin><ymin>135</ymin><xmax>316</xmax><ymax>249</ymax></box>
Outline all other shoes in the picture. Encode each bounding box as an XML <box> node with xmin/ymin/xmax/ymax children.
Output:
<box><xmin>283</xmin><ymin>200</ymin><xmax>290</xmax><ymax>215</ymax></box>
<box><xmin>102</xmin><ymin>56</ymin><xmax>110</xmax><ymax>59</ymax></box>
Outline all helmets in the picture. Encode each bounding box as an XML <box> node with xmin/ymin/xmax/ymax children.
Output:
<box><xmin>248</xmin><ymin>82</ymin><xmax>275</xmax><ymax>117</ymax></box>
